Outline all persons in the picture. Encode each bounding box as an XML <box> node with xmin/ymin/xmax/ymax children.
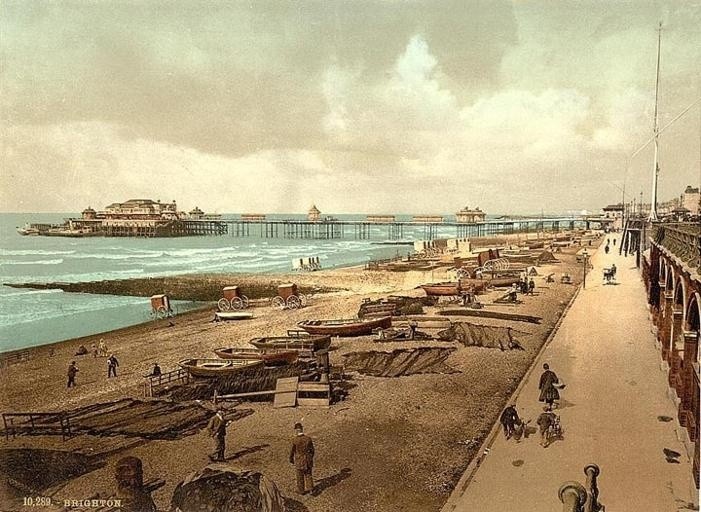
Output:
<box><xmin>289</xmin><ymin>423</ymin><xmax>317</xmax><ymax>495</ymax></box>
<box><xmin>500</xmin><ymin>403</ymin><xmax>522</xmax><ymax>436</ymax></box>
<box><xmin>535</xmin><ymin>407</ymin><xmax>555</xmax><ymax>448</ymax></box>
<box><xmin>505</xmin><ymin>276</ymin><xmax>535</xmax><ymax>301</ymax></box>
<box><xmin>66</xmin><ymin>338</ymin><xmax>119</xmax><ymax>387</ymax></box>
<box><xmin>206</xmin><ymin>408</ymin><xmax>228</xmax><ymax>462</ymax></box>
<box><xmin>538</xmin><ymin>363</ymin><xmax>559</xmax><ymax>411</ymax></box>
<box><xmin>611</xmin><ymin>264</ymin><xmax>616</xmax><ymax>279</ymax></box>
<box><xmin>152</xmin><ymin>362</ymin><xmax>161</xmax><ymax>375</ymax></box>
